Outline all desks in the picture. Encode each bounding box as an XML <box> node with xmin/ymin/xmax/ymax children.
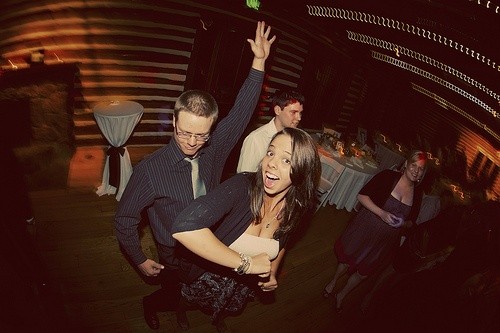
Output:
<box><xmin>92</xmin><ymin>100</ymin><xmax>145</xmax><ymax>200</ymax></box>
<box><xmin>319</xmin><ymin>141</ymin><xmax>406</xmax><ymax>212</ymax></box>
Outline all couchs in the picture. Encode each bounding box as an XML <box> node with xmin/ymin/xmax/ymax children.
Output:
<box><xmin>0</xmin><ymin>80</ymin><xmax>76</xmax><ymax>189</ymax></box>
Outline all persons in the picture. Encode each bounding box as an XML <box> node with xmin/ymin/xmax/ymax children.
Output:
<box><xmin>415</xmin><ymin>186</ymin><xmax>454</xmax><ymax>226</ymax></box>
<box><xmin>114</xmin><ymin>20</ymin><xmax>277</xmax><ymax>333</ymax></box>
<box><xmin>141</xmin><ymin>126</ymin><xmax>322</xmax><ymax>333</ymax></box>
<box><xmin>235</xmin><ymin>87</ymin><xmax>305</xmax><ymax>173</ymax></box>
<box><xmin>318</xmin><ymin>149</ymin><xmax>429</xmax><ymax>315</ymax></box>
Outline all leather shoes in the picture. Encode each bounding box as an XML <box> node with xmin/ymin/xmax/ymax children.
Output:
<box><xmin>143</xmin><ymin>296</ymin><xmax>160</xmax><ymax>330</ymax></box>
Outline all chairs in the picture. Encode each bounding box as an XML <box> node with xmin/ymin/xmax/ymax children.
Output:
<box><xmin>315</xmin><ymin>155</ymin><xmax>347</xmax><ymax>215</ymax></box>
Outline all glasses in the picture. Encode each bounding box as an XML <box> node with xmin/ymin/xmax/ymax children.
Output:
<box><xmin>175</xmin><ymin>126</ymin><xmax>209</xmax><ymax>142</ymax></box>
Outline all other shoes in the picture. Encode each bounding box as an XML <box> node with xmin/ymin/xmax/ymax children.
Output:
<box><xmin>323</xmin><ymin>289</ymin><xmax>336</xmax><ymax>297</ymax></box>
<box><xmin>334</xmin><ymin>294</ymin><xmax>345</xmax><ymax>315</ymax></box>
<box><xmin>176</xmin><ymin>310</ymin><xmax>190</xmax><ymax>329</ymax></box>
<box><xmin>212</xmin><ymin>319</ymin><xmax>227</xmax><ymax>333</ymax></box>
